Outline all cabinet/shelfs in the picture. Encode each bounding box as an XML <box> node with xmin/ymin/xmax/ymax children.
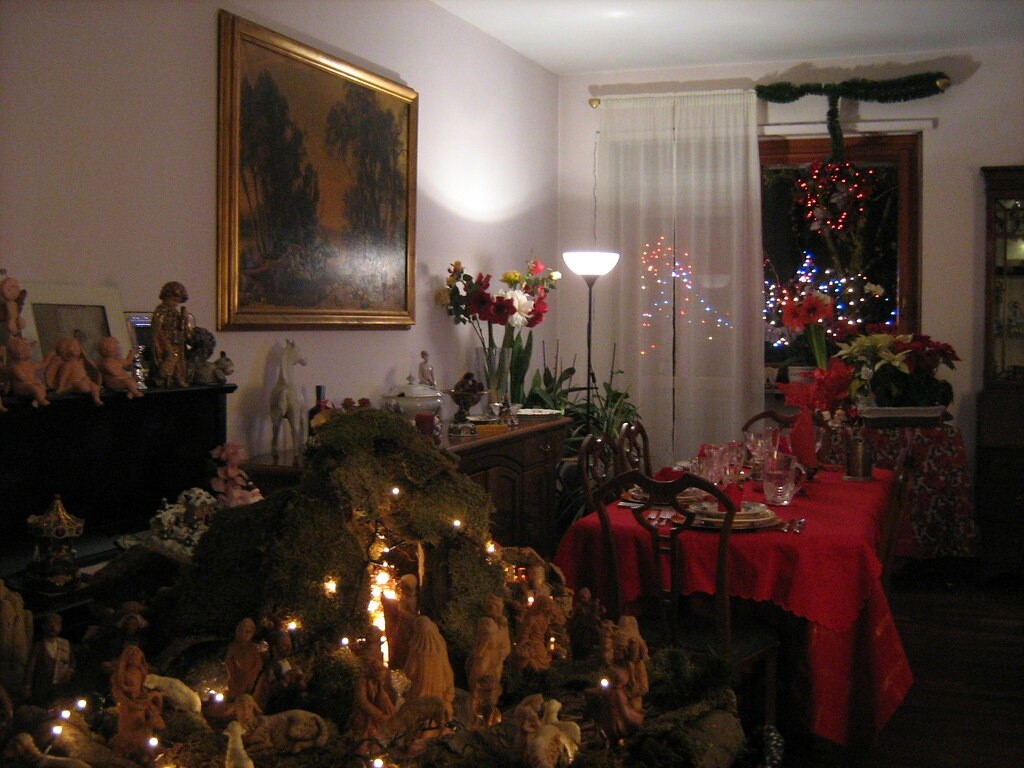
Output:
<box><xmin>976</xmin><ymin>164</ymin><xmax>1024</xmax><ymax>484</ymax></box>
<box><xmin>457</xmin><ymin>425</ymin><xmax>569</xmax><ymax>562</ymax></box>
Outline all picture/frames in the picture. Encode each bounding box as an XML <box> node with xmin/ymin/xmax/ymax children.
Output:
<box><xmin>214</xmin><ymin>7</ymin><xmax>419</xmax><ymax>331</ymax></box>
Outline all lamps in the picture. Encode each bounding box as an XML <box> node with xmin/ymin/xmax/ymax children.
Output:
<box><xmin>561</xmin><ymin>250</ymin><xmax>621</xmax><ymax>477</ymax></box>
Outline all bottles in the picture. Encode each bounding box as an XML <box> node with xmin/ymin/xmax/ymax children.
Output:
<box><xmin>308</xmin><ymin>386</ymin><xmax>330</xmax><ymax>437</ymax></box>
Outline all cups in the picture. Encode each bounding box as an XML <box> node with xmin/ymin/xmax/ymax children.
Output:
<box><xmin>765</xmin><ymin>455</ymin><xmax>806</xmax><ymax>506</ymax></box>
<box><xmin>690</xmin><ymin>427</ymin><xmax>779</xmax><ymax>484</ymax></box>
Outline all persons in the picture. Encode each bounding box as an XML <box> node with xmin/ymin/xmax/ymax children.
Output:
<box><xmin>519</xmin><ymin>596</ymin><xmax>566</xmax><ymax>671</ymax></box>
<box><xmin>52</xmin><ymin>340</ymin><xmax>104</xmax><ymax>407</ymax></box>
<box><xmin>24</xmin><ymin>610</ymin><xmax>78</xmax><ymax>700</ymax></box>
<box><xmin>345</xmin><ymin>659</ymin><xmax>396</xmax><ymax>755</ymax></box>
<box><xmin>0</xmin><ymin>540</ymin><xmax>648</xmax><ymax>768</ymax></box>
<box><xmin>148</xmin><ymin>281</ymin><xmax>193</xmax><ymax>387</ymax></box>
<box><xmin>188</xmin><ymin>327</ymin><xmax>228</xmax><ymax>386</ymax></box>
<box><xmin>418</xmin><ymin>351</ymin><xmax>436</xmax><ymax>387</ymax></box>
<box><xmin>399</xmin><ymin>615</ymin><xmax>455</xmax><ymax>736</ymax></box>
<box><xmin>251</xmin><ymin>631</ymin><xmax>310</xmax><ymax>716</ymax></box>
<box><xmin>114</xmin><ymin>646</ymin><xmax>162</xmax><ymax>749</ymax></box>
<box><xmin>466</xmin><ymin>592</ymin><xmax>510</xmax><ymax>727</ymax></box>
<box><xmin>97</xmin><ymin>337</ymin><xmax>144</xmax><ymax>399</ymax></box>
<box><xmin>8</xmin><ymin>337</ymin><xmax>56</xmax><ymax>407</ymax></box>
<box><xmin>501</xmin><ymin>388</ymin><xmax>511</xmax><ymax>409</ymax></box>
<box><xmin>68</xmin><ymin>324</ymin><xmax>102</xmax><ymax>363</ymax></box>
<box><xmin>210</xmin><ymin>440</ymin><xmax>265</xmax><ymax>508</ymax></box>
<box><xmin>570</xmin><ymin>582</ymin><xmax>606</xmax><ymax>659</ymax></box>
<box><xmin>0</xmin><ymin>276</ymin><xmax>27</xmax><ymax>413</ymax></box>
<box><xmin>224</xmin><ymin>618</ymin><xmax>263</xmax><ymax>694</ymax></box>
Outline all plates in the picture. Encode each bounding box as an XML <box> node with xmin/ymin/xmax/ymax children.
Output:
<box><xmin>517</xmin><ymin>409</ymin><xmax>561</xmax><ymax>421</ymax></box>
<box><xmin>644</xmin><ymin>490</ymin><xmax>781</xmax><ymax>529</ymax></box>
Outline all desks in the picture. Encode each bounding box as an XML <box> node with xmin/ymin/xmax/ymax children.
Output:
<box><xmin>821</xmin><ymin>419</ymin><xmax>980</xmax><ymax>589</ymax></box>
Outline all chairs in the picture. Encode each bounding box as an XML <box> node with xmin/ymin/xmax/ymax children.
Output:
<box><xmin>591</xmin><ymin>466</ymin><xmax>782</xmax><ymax>728</ymax></box>
<box><xmin>742</xmin><ymin>411</ymin><xmax>832</xmax><ymax>466</ymax></box>
<box><xmin>579</xmin><ymin>430</ymin><xmax>619</xmax><ymax>513</ymax></box>
<box><xmin>618</xmin><ymin>420</ymin><xmax>652</xmax><ymax>501</ymax></box>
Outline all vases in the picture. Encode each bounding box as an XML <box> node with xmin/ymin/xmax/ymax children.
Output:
<box><xmin>871</xmin><ymin>370</ymin><xmax>942</xmax><ymax>409</ymax></box>
<box><xmin>474</xmin><ymin>346</ymin><xmax>519</xmax><ymax>426</ymax></box>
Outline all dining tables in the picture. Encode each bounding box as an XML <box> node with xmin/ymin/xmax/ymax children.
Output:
<box><xmin>556</xmin><ymin>468</ymin><xmax>896</xmax><ymax>751</ymax></box>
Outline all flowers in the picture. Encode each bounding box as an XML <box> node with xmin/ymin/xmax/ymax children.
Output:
<box><xmin>433</xmin><ymin>258</ymin><xmax>562</xmax><ymax>411</ymax></box>
<box><xmin>779</xmin><ymin>286</ymin><xmax>963</xmax><ymax>440</ymax></box>
